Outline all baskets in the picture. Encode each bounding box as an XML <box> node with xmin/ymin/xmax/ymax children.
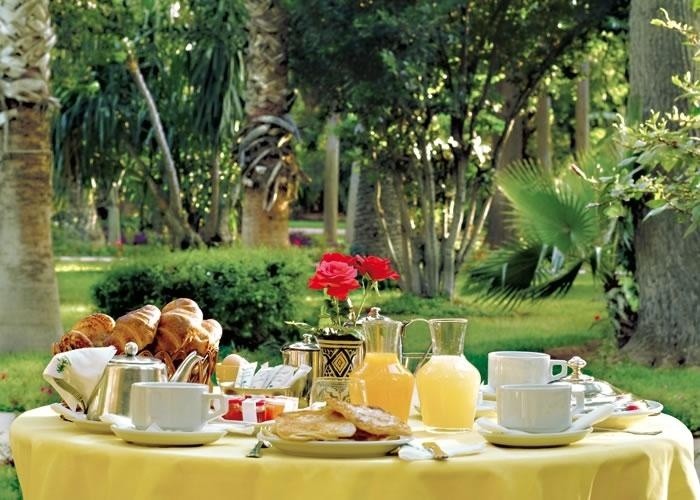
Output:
<box><xmin>53</xmin><ymin>336</ymin><xmax>218</xmax><ymax>383</ymax></box>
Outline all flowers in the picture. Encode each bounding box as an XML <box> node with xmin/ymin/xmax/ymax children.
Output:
<box><xmin>308</xmin><ymin>250</ymin><xmax>400</xmax><ymax>341</ymax></box>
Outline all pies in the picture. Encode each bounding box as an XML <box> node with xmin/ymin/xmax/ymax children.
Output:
<box><xmin>274</xmin><ymin>396</ymin><xmax>411</xmax><ymax>440</ymax></box>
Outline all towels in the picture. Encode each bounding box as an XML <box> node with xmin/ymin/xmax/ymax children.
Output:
<box><xmin>42</xmin><ymin>344</ymin><xmax>117</xmax><ymax>413</ymax></box>
<box><xmin>396</xmin><ymin>435</ymin><xmax>486</xmax><ymax>461</ymax></box>
<box><xmin>474</xmin><ymin>403</ymin><xmax>615</xmax><ymax>437</ymax></box>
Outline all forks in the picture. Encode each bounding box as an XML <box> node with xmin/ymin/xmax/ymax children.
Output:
<box><xmin>248</xmin><ymin>425</ymin><xmax>271</xmax><ymax>457</ymax></box>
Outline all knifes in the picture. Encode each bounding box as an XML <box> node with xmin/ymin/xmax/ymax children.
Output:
<box><xmin>423</xmin><ymin>441</ymin><xmax>449</xmax><ymax>460</ymax></box>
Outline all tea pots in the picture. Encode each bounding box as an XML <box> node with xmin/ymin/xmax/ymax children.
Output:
<box><xmin>280</xmin><ymin>333</ymin><xmax>320</xmax><ymax>386</ymax></box>
<box><xmin>53</xmin><ymin>341</ymin><xmax>205</xmax><ymax>422</ymax></box>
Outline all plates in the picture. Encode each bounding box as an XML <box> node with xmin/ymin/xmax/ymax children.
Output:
<box><xmin>474</xmin><ymin>399</ymin><xmax>663</xmax><ymax>448</ymax></box>
<box><xmin>220</xmin><ymin>416</ymin><xmax>407</xmax><ymax>458</ymax></box>
<box><xmin>63</xmin><ymin>413</ymin><xmax>230</xmax><ymax>446</ymax></box>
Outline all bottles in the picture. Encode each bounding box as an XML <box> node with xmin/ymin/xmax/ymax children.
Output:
<box><xmin>348</xmin><ymin>319</ymin><xmax>481</xmax><ymax>433</ymax></box>
<box><xmin>223</xmin><ymin>396</ymin><xmax>265</xmax><ymax>422</ymax></box>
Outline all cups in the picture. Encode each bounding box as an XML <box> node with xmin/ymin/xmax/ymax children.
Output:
<box><xmin>488</xmin><ymin>351</ymin><xmax>586</xmax><ymax>434</ymax></box>
<box><xmin>131</xmin><ymin>379</ymin><xmax>230</xmax><ymax>432</ymax></box>
<box><xmin>309</xmin><ymin>377</ymin><xmax>367</xmax><ymax>406</ymax></box>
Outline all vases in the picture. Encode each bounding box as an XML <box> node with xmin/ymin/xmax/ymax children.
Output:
<box><xmin>313</xmin><ymin>333</ymin><xmax>363</xmax><ymax>404</ymax></box>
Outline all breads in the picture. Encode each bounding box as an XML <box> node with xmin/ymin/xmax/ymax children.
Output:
<box><xmin>58</xmin><ymin>298</ymin><xmax>223</xmax><ymax>360</ymax></box>
